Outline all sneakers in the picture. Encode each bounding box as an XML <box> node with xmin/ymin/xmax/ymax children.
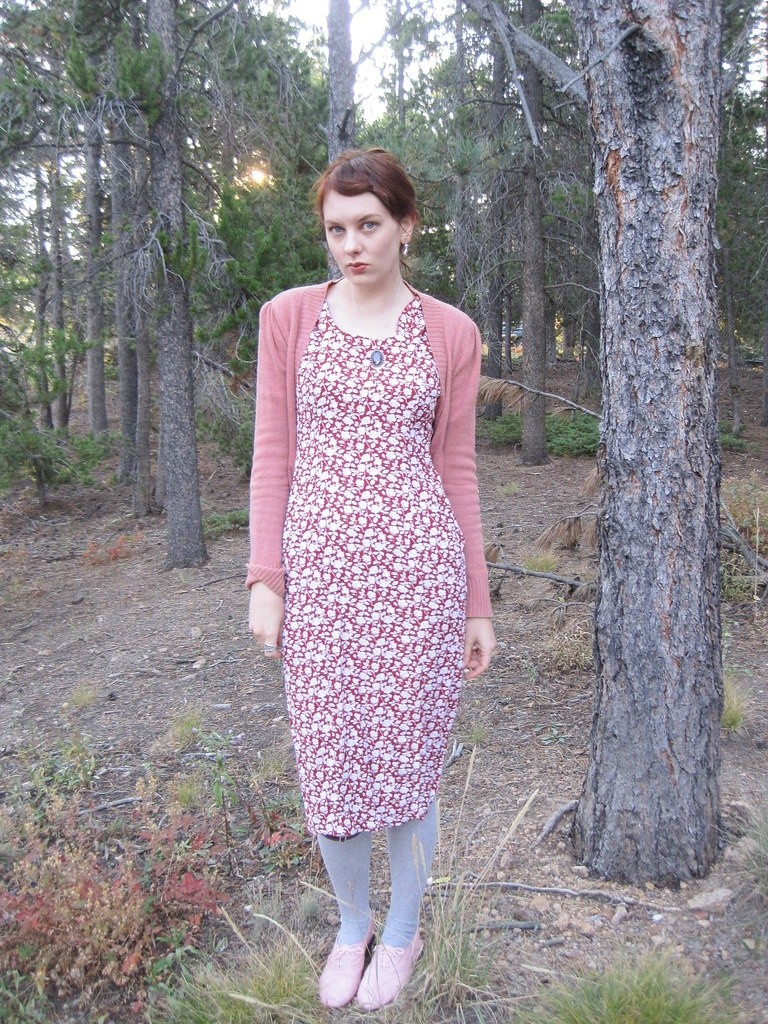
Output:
<box><xmin>357</xmin><ymin>928</ymin><xmax>424</xmax><ymax>1011</ymax></box>
<box><xmin>318</xmin><ymin>908</ymin><xmax>375</xmax><ymax>1008</ymax></box>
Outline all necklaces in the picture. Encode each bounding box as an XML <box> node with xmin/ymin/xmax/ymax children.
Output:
<box><xmin>346</xmin><ymin>277</ymin><xmax>402</xmax><ymax>368</ymax></box>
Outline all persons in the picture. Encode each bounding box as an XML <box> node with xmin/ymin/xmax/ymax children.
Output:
<box><xmin>247</xmin><ymin>150</ymin><xmax>498</xmax><ymax>1009</ymax></box>
